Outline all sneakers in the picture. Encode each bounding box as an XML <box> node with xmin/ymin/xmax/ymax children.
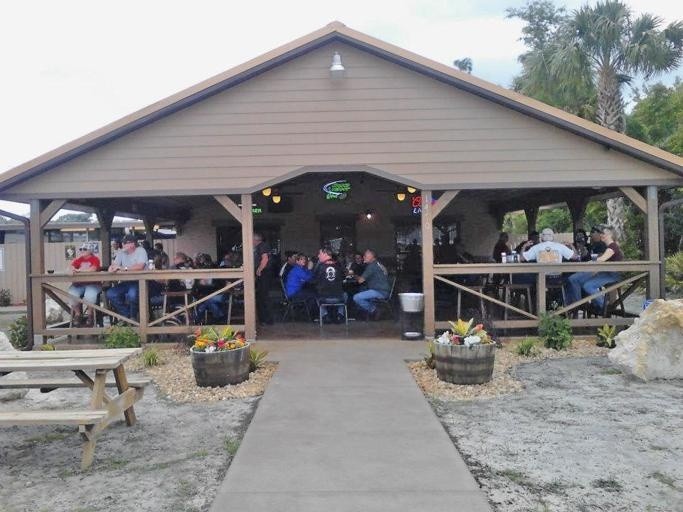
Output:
<box><xmin>85</xmin><ymin>318</ymin><xmax>94</xmax><ymax>327</ymax></box>
<box><xmin>335</xmin><ymin>315</ymin><xmax>343</xmax><ymax>323</ymax></box>
<box><xmin>313</xmin><ymin>316</ymin><xmax>326</xmax><ymax>322</ymax></box>
<box><xmin>70</xmin><ymin>315</ymin><xmax>82</xmax><ymax>323</ymax></box>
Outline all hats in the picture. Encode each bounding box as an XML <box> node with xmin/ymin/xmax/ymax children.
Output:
<box><xmin>541</xmin><ymin>228</ymin><xmax>552</xmax><ymax>241</ymax></box>
<box><xmin>119</xmin><ymin>235</ymin><xmax>137</xmax><ymax>243</ymax></box>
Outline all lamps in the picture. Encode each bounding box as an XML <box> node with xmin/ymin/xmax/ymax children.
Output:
<box><xmin>396</xmin><ymin>192</ymin><xmax>405</xmax><ymax>201</ymax></box>
<box><xmin>407</xmin><ymin>186</ymin><xmax>416</xmax><ymax>194</ymax></box>
<box><xmin>272</xmin><ymin>187</ymin><xmax>282</xmax><ymax>204</ymax></box>
<box><xmin>262</xmin><ymin>187</ymin><xmax>272</xmax><ymax>197</ymax></box>
<box><xmin>330</xmin><ymin>51</ymin><xmax>345</xmax><ymax>72</ymax></box>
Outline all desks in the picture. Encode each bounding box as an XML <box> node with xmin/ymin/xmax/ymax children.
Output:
<box><xmin>0</xmin><ymin>348</ymin><xmax>142</xmax><ymax>473</ymax></box>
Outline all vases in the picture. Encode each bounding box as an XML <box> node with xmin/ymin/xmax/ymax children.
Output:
<box><xmin>190</xmin><ymin>346</ymin><xmax>251</xmax><ymax>386</ymax></box>
<box><xmin>434</xmin><ymin>339</ymin><xmax>496</xmax><ymax>383</ymax></box>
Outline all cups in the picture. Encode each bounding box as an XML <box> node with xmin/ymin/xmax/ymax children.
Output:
<box><xmin>590</xmin><ymin>253</ymin><xmax>599</xmax><ymax>263</ymax></box>
<box><xmin>507</xmin><ymin>255</ymin><xmax>517</xmax><ymax>263</ymax></box>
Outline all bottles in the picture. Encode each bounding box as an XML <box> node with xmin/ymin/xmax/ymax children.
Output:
<box><xmin>148</xmin><ymin>259</ymin><xmax>153</xmax><ymax>270</ymax></box>
<box><xmin>501</xmin><ymin>251</ymin><xmax>507</xmax><ymax>264</ymax></box>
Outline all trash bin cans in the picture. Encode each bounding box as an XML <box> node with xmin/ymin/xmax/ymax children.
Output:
<box><xmin>397</xmin><ymin>293</ymin><xmax>425</xmax><ymax>341</ymax></box>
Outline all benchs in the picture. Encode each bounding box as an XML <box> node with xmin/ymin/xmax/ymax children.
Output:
<box><xmin>0</xmin><ymin>379</ymin><xmax>151</xmax><ymax>400</ymax></box>
<box><xmin>0</xmin><ymin>411</ymin><xmax>108</xmax><ymax>442</ymax></box>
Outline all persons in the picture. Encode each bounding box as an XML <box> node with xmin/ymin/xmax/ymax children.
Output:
<box><xmin>106</xmin><ymin>234</ymin><xmax>245</xmax><ymax>327</ymax></box>
<box><xmin>251</xmin><ymin>232</ymin><xmax>391</xmax><ymax>326</ymax></box>
<box><xmin>68</xmin><ymin>242</ymin><xmax>103</xmax><ymax>326</ymax></box>
<box><xmin>492</xmin><ymin>225</ymin><xmax>624</xmax><ymax>315</ymax></box>
<box><xmin>404</xmin><ymin>236</ymin><xmax>479</xmax><ymax>281</ymax></box>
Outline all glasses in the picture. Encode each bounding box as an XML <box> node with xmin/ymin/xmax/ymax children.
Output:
<box><xmin>78</xmin><ymin>248</ymin><xmax>87</xmax><ymax>251</ymax></box>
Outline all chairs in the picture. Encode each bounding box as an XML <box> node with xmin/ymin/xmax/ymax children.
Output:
<box><xmin>70</xmin><ymin>257</ymin><xmax>631</xmax><ymax>342</ymax></box>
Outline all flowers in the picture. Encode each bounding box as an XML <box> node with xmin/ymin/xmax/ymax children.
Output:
<box><xmin>437</xmin><ymin>318</ymin><xmax>494</xmax><ymax>344</ymax></box>
<box><xmin>193</xmin><ymin>326</ymin><xmax>246</xmax><ymax>352</ymax></box>
